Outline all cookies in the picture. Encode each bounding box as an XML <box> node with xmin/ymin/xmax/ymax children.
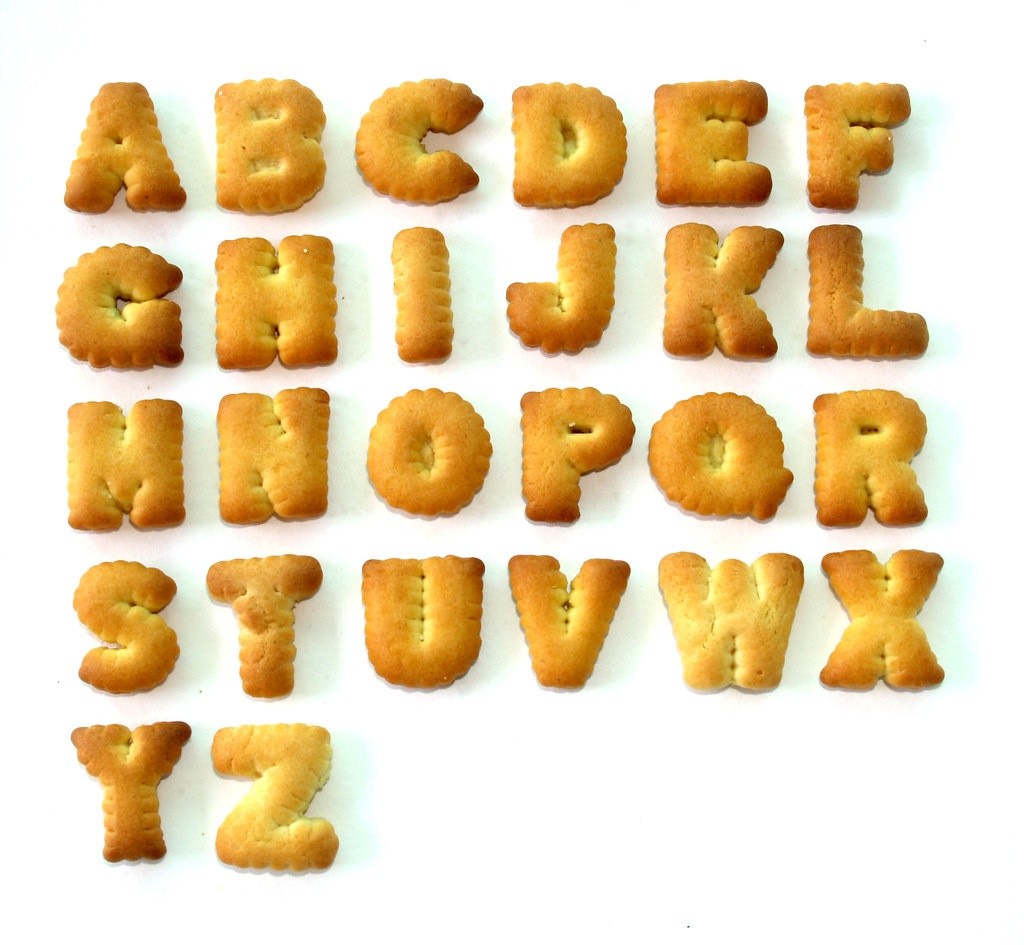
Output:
<box><xmin>60</xmin><ymin>76</ymin><xmax>946</xmax><ymax>872</ymax></box>
